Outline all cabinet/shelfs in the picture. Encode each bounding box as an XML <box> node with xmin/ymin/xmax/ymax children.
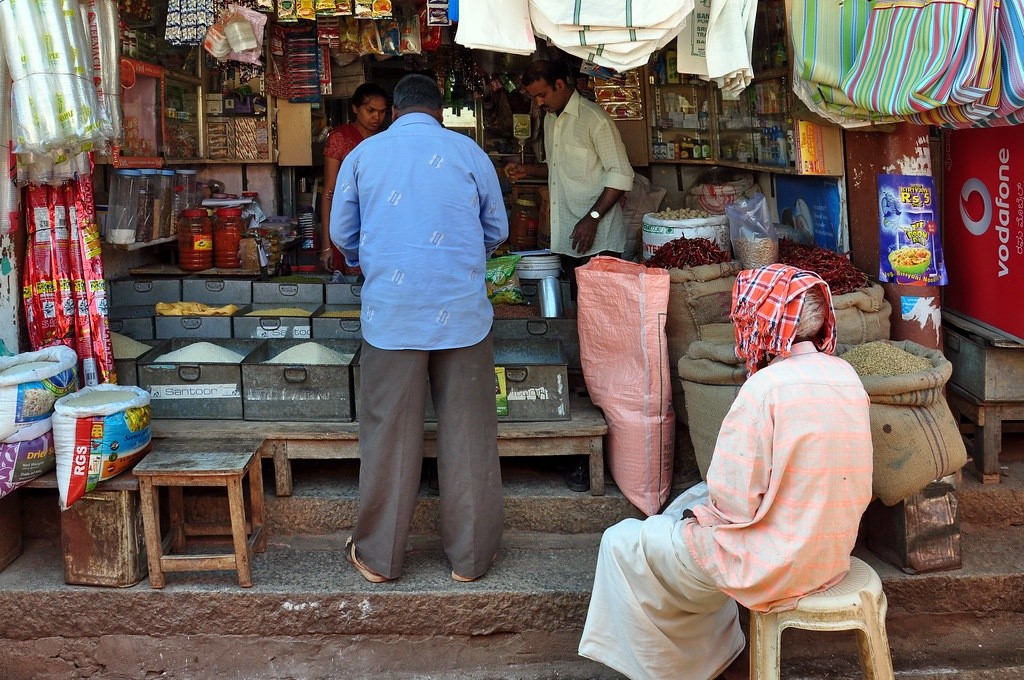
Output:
<box><xmin>91</xmin><ymin>0</ymin><xmax>365</xmax><ymax>168</ymax></box>
<box><xmin>593</xmin><ymin>0</ymin><xmax>845</xmax><ymax>177</ymax></box>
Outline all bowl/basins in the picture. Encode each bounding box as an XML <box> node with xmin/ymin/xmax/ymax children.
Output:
<box><xmin>888</xmin><ymin>248</ymin><xmax>931</xmax><ymax>274</ymax></box>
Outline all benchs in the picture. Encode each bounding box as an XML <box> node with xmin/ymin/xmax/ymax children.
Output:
<box><xmin>0</xmin><ymin>478</ymin><xmax>148</xmax><ymax>586</ymax></box>
<box><xmin>149</xmin><ymin>383</ymin><xmax>610</xmax><ymax>495</ymax></box>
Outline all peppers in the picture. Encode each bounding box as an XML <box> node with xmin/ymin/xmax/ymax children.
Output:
<box><xmin>778</xmin><ymin>235</ymin><xmax>871</xmax><ymax>295</ymax></box>
<box><xmin>642</xmin><ymin>231</ymin><xmax>729</xmax><ymax>269</ymax></box>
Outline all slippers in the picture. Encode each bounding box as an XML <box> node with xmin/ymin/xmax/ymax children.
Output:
<box><xmin>345</xmin><ymin>536</ymin><xmax>387</xmax><ymax>582</ymax></box>
<box><xmin>451</xmin><ymin>551</ymin><xmax>497</xmax><ymax>582</ymax></box>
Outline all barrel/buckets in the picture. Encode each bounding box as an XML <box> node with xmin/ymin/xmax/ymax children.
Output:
<box><xmin>514</xmin><ymin>255</ymin><xmax>564</xmax><ymax>305</ymax></box>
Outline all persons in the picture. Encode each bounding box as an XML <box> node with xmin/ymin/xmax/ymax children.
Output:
<box><xmin>505</xmin><ymin>60</ymin><xmax>636</xmax><ymax>290</ymax></box>
<box><xmin>327</xmin><ymin>74</ymin><xmax>509</xmax><ymax>582</ymax></box>
<box><xmin>321</xmin><ymin>82</ymin><xmax>388</xmax><ymax>276</ymax></box>
<box><xmin>578</xmin><ymin>261</ymin><xmax>875</xmax><ymax>680</ymax></box>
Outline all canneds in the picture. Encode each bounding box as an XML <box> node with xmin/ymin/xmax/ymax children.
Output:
<box><xmin>508</xmin><ymin>198</ymin><xmax>538</xmax><ymax>247</ymax></box>
<box><xmin>211</xmin><ymin>191</ymin><xmax>259</xmax><ymax>199</ymax></box>
<box><xmin>652</xmin><ymin>142</ymin><xmax>667</xmax><ymax>160</ymax></box>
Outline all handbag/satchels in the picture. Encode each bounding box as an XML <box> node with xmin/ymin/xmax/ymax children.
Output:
<box><xmin>724</xmin><ymin>193</ymin><xmax>779</xmax><ymax>270</ymax></box>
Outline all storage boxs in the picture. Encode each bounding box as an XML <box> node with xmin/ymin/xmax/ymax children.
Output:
<box><xmin>106</xmin><ymin>276</ymin><xmax>569</xmax><ymax>423</ymax></box>
<box><xmin>943</xmin><ymin>326</ymin><xmax>1024</xmax><ymax>405</ymax></box>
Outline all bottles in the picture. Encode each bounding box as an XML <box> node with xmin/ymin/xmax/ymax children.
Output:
<box><xmin>105</xmin><ymin>168</ymin><xmax>143</xmax><ymax>245</ymax></box>
<box><xmin>170</xmin><ymin>194</ymin><xmax>180</xmax><ymax>235</ymax></box>
<box><xmin>215</xmin><ymin>207</ymin><xmax>245</xmax><ymax>269</ymax></box>
<box><xmin>136</xmin><ymin>168</ymin><xmax>158</xmax><ymax>243</ymax></box>
<box><xmin>508</xmin><ymin>197</ymin><xmax>538</xmax><ymax>247</ymax></box>
<box><xmin>722</xmin><ymin>118</ymin><xmax>795</xmax><ymax>167</ymax></box>
<box><xmin>752</xmin><ymin>41</ymin><xmax>788</xmax><ymax>70</ymax></box>
<box><xmin>175</xmin><ymin>169</ymin><xmax>197</xmax><ymax>212</ymax></box>
<box><xmin>723</xmin><ymin>76</ymin><xmax>791</xmax><ymax>115</ymax></box>
<box><xmin>653</xmin><ymin>47</ymin><xmax>706</xmax><ymax>84</ymax></box>
<box><xmin>670</xmin><ymin>98</ymin><xmax>711</xmax><ymax>159</ymax></box>
<box><xmin>177</xmin><ymin>209</ymin><xmax>213</xmax><ymax>271</ymax></box>
<box><xmin>153</xmin><ymin>170</ymin><xmax>175</xmax><ymax>240</ymax></box>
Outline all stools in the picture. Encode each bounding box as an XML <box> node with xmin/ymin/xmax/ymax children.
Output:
<box><xmin>133</xmin><ymin>438</ymin><xmax>268</xmax><ymax>588</ymax></box>
<box><xmin>750</xmin><ymin>556</ymin><xmax>895</xmax><ymax>680</ymax></box>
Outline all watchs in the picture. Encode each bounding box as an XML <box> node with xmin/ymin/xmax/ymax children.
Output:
<box><xmin>588</xmin><ymin>209</ymin><xmax>602</xmax><ymax>223</ymax></box>
